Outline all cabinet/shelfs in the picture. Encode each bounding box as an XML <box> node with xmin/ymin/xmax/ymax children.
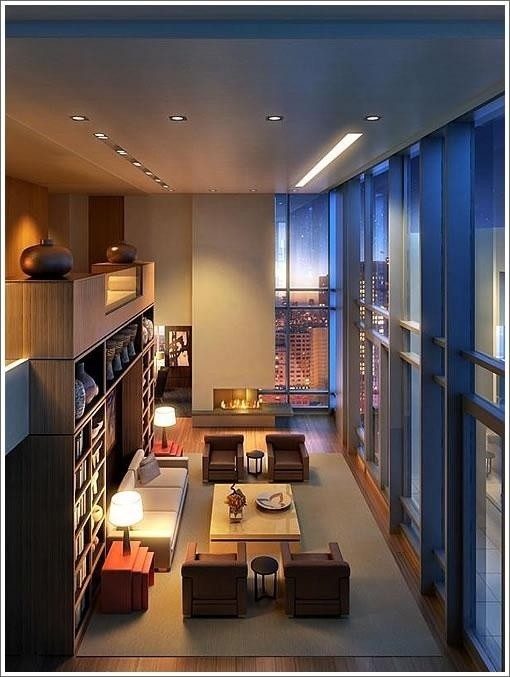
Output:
<box><xmin>27</xmin><ymin>305</ymin><xmax>154</xmax><ymax>434</ymax></box>
<box><xmin>5</xmin><ymin>262</ymin><xmax>155</xmax><ymax>357</ymax></box>
<box><xmin>121</xmin><ymin>343</ymin><xmax>156</xmax><ymax>474</ymax></box>
<box><xmin>5</xmin><ymin>404</ymin><xmax>108</xmax><ymax>660</ymax></box>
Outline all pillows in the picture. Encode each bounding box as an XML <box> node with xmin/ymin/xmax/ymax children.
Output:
<box><xmin>137</xmin><ymin>461</ymin><xmax>161</xmax><ymax>484</ymax></box>
<box><xmin>107</xmin><ymin>276</ymin><xmax>136</xmax><ymax>291</ymax></box>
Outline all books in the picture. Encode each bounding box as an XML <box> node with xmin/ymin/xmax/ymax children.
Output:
<box><xmin>75</xmin><ymin>421</ymin><xmax>90</xmax><ymax>630</ymax></box>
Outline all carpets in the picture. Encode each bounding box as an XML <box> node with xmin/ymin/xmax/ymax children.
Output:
<box><xmin>76</xmin><ymin>453</ymin><xmax>442</xmax><ymax>657</ymax></box>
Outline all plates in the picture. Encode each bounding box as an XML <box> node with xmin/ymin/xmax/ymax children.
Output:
<box><xmin>256</xmin><ymin>490</ymin><xmax>293</xmax><ymax>510</ymax></box>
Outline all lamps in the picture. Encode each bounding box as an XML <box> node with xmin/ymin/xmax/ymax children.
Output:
<box><xmin>153</xmin><ymin>406</ymin><xmax>177</xmax><ymax>448</ymax></box>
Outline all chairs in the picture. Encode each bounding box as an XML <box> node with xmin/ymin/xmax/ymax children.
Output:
<box><xmin>181</xmin><ymin>542</ymin><xmax>250</xmax><ymax>620</ymax></box>
<box><xmin>266</xmin><ymin>434</ymin><xmax>310</xmax><ymax>484</ymax></box>
<box><xmin>279</xmin><ymin>541</ymin><xmax>350</xmax><ymax>622</ymax></box>
<box><xmin>155</xmin><ymin>366</ymin><xmax>170</xmax><ymax>401</ymax></box>
<box><xmin>201</xmin><ymin>434</ymin><xmax>247</xmax><ymax>485</ymax></box>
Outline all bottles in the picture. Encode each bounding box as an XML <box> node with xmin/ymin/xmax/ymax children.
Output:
<box><xmin>77</xmin><ymin>362</ymin><xmax>98</xmax><ymax>403</ymax></box>
<box><xmin>108</xmin><ymin>239</ymin><xmax>138</xmax><ymax>262</ymax></box>
<box><xmin>75</xmin><ymin>379</ymin><xmax>87</xmax><ymax>418</ymax></box>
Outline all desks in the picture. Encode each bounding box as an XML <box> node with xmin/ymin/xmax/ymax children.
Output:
<box><xmin>151</xmin><ymin>440</ymin><xmax>184</xmax><ymax>457</ymax></box>
<box><xmin>246</xmin><ymin>449</ymin><xmax>265</xmax><ymax>479</ymax></box>
<box><xmin>250</xmin><ymin>556</ymin><xmax>278</xmax><ymax>604</ymax></box>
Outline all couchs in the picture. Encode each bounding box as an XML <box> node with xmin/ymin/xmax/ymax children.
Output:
<box><xmin>105</xmin><ymin>449</ymin><xmax>192</xmax><ymax>572</ymax></box>
